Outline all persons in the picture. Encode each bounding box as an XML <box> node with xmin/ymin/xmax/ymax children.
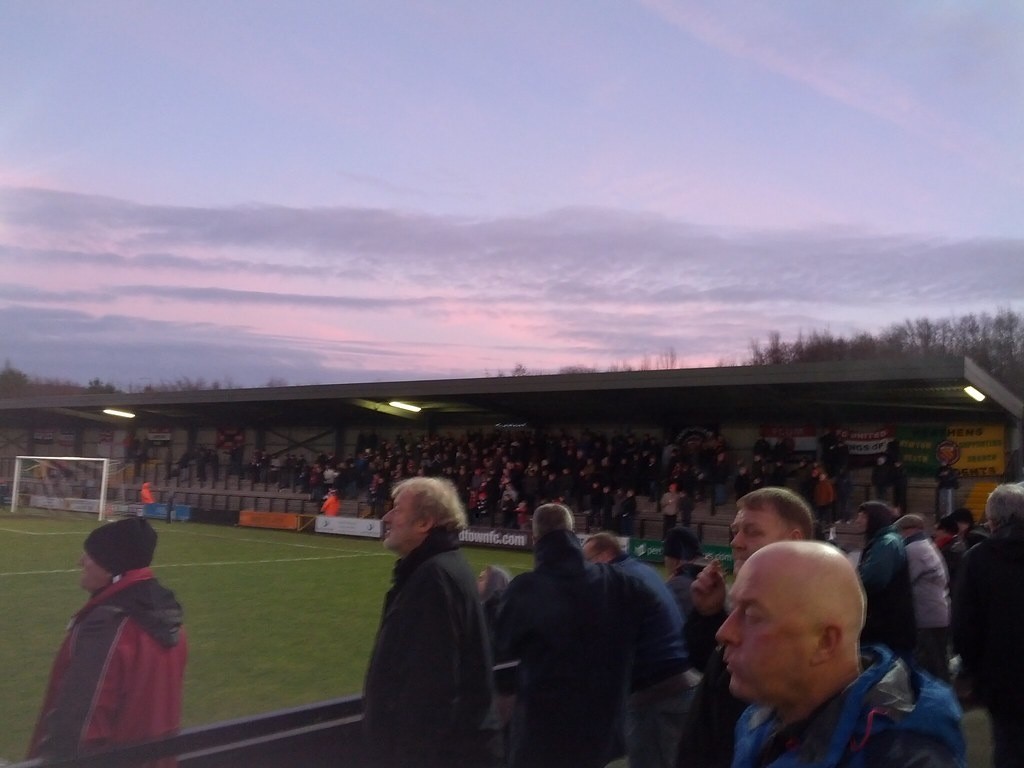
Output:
<box><xmin>321</xmin><ymin>489</ymin><xmax>340</xmax><ymax>517</ymax></box>
<box><xmin>365</xmin><ymin>477</ymin><xmax>494</xmax><ymax>766</ymax></box>
<box><xmin>715</xmin><ymin>539</ymin><xmax>972</xmax><ymax>768</ymax></box>
<box><xmin>26</xmin><ymin>516</ymin><xmax>189</xmax><ymax>768</ymax></box>
<box><xmin>933</xmin><ymin>460</ymin><xmax>959</xmax><ymax>526</ymax></box>
<box><xmin>179</xmin><ymin>423</ymin><xmax>907</xmax><ymax>543</ymax></box>
<box><xmin>472</xmin><ymin>480</ymin><xmax>1024</xmax><ymax>768</ymax></box>
<box><xmin>142</xmin><ymin>482</ymin><xmax>155</xmax><ymax>504</ymax></box>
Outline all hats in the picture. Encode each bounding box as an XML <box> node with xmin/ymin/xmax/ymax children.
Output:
<box><xmin>82</xmin><ymin>516</ymin><xmax>158</xmax><ymax>575</ymax></box>
<box><xmin>663</xmin><ymin>526</ymin><xmax>701</xmax><ymax>561</ymax></box>
<box><xmin>894</xmin><ymin>515</ymin><xmax>924</xmax><ymax>531</ymax></box>
